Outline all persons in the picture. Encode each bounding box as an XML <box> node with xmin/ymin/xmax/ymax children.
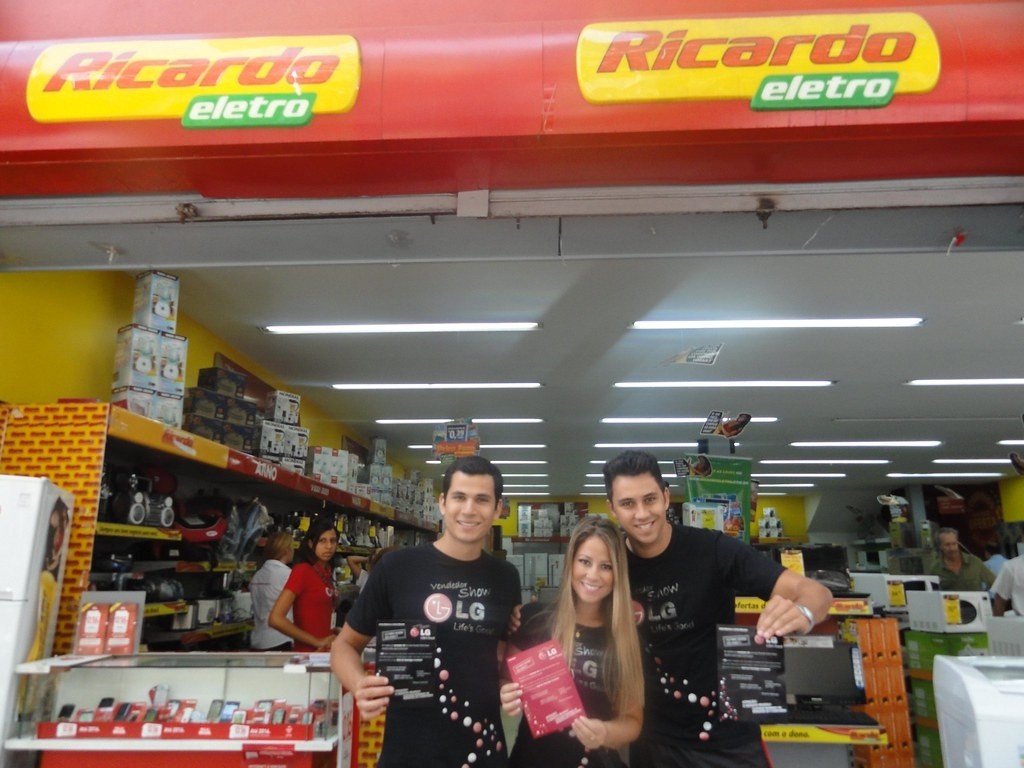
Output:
<box><xmin>268</xmin><ymin>519</ymin><xmax>342</xmax><ymax>652</ymax></box>
<box><xmin>982</xmin><ymin>540</ymin><xmax>1013</xmax><ymax>611</ymax></box>
<box><xmin>687</xmin><ymin>456</ymin><xmax>711</xmax><ymax>477</ymax></box>
<box><xmin>989</xmin><ymin>550</ymin><xmax>1024</xmax><ymax>616</ymax></box>
<box><xmin>347</xmin><ymin>547</ymin><xmax>403</xmax><ymax>594</ymax></box>
<box><xmin>498</xmin><ymin>516</ymin><xmax>645</xmax><ymax>768</ymax></box>
<box><xmin>507</xmin><ymin>450</ymin><xmax>833</xmax><ymax>768</ymax></box>
<box><xmin>248</xmin><ymin>531</ymin><xmax>295</xmax><ymax>651</ymax></box>
<box><xmin>718</xmin><ymin>414</ymin><xmax>751</xmax><ymax>437</ymax></box>
<box><xmin>928</xmin><ymin>527</ymin><xmax>997</xmax><ymax>591</ymax></box>
<box><xmin>329</xmin><ymin>456</ymin><xmax>521</xmax><ymax>768</ymax></box>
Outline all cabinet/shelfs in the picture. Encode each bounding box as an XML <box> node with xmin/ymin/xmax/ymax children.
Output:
<box><xmin>0</xmin><ymin>400</ymin><xmax>439</xmax><ymax>661</ymax></box>
<box><xmin>732</xmin><ymin>596</ymin><xmax>890</xmax><ymax>747</ymax></box>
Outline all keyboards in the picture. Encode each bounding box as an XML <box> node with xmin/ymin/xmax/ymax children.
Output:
<box><xmin>760</xmin><ymin>711</ymin><xmax>879</xmax><ymax>725</ymax></box>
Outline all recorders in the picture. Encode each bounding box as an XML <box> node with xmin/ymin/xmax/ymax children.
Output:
<box><xmin>112</xmin><ymin>492</ymin><xmax>175</xmax><ymax>527</ymax></box>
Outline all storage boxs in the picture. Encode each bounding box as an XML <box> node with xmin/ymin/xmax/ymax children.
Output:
<box><xmin>40</xmin><ymin>269</ymin><xmax>993</xmax><ymax>768</ymax></box>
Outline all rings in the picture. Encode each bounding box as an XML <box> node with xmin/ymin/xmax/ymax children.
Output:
<box><xmin>590</xmin><ymin>736</ymin><xmax>595</xmax><ymax>740</ymax></box>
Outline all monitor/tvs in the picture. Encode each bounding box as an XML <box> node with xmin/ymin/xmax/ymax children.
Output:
<box><xmin>784</xmin><ymin>641</ymin><xmax>868</xmax><ymax>711</ymax></box>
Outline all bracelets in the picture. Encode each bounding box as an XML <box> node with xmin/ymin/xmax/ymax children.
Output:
<box><xmin>794</xmin><ymin>604</ymin><xmax>813</xmax><ymax>635</ymax></box>
